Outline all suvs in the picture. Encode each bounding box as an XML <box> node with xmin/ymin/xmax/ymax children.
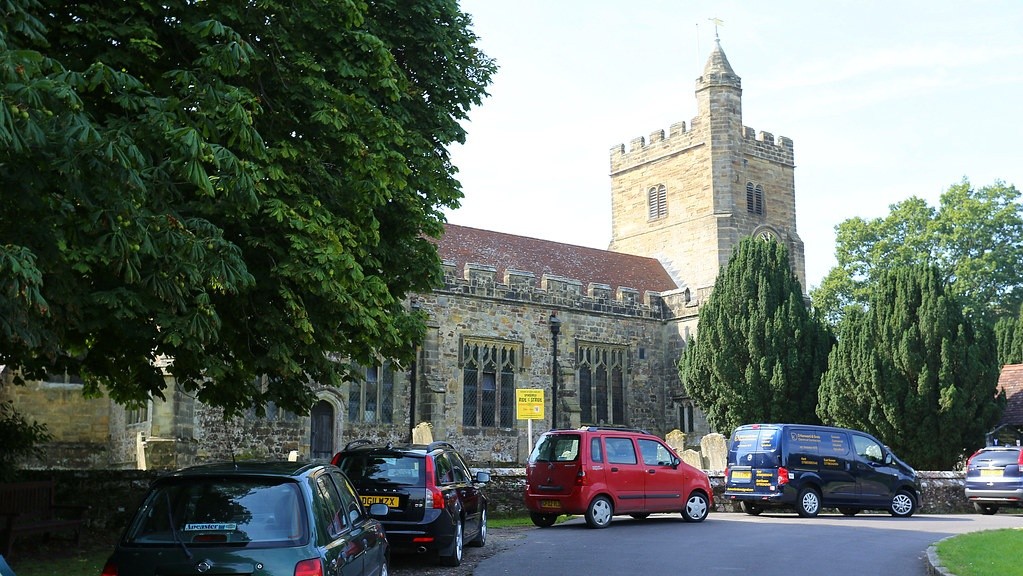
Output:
<box><xmin>524</xmin><ymin>425</ymin><xmax>712</xmax><ymax>528</ymax></box>
<box><xmin>329</xmin><ymin>440</ymin><xmax>490</xmax><ymax>569</ymax></box>
<box><xmin>102</xmin><ymin>419</ymin><xmax>389</xmax><ymax>576</ymax></box>
<box><xmin>964</xmin><ymin>439</ymin><xmax>1023</xmax><ymax>515</ymax></box>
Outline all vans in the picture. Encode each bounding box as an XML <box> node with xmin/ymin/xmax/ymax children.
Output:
<box><xmin>725</xmin><ymin>425</ymin><xmax>923</xmax><ymax>518</ymax></box>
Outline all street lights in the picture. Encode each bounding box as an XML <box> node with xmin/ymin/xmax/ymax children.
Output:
<box><xmin>548</xmin><ymin>314</ymin><xmax>561</xmax><ymax>432</ymax></box>
